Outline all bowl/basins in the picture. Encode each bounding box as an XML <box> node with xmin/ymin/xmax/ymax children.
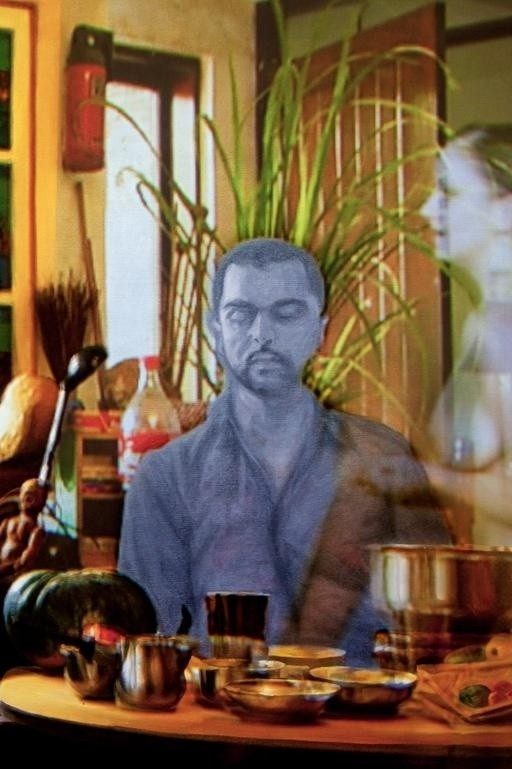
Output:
<box><xmin>265</xmin><ymin>644</ymin><xmax>346</xmax><ymax>676</ymax></box>
<box><xmin>192</xmin><ymin>661</ymin><xmax>282</xmax><ymax>706</ymax></box>
<box><xmin>314</xmin><ymin>664</ymin><xmax>419</xmax><ymax>712</ymax></box>
<box><xmin>225</xmin><ymin>680</ymin><xmax>341</xmax><ymax>718</ymax></box>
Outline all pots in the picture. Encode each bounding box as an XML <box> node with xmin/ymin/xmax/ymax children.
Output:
<box><xmin>375</xmin><ymin>546</ymin><xmax>512</xmax><ymax>670</ymax></box>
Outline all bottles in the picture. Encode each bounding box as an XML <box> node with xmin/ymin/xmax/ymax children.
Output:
<box><xmin>116</xmin><ymin>355</ymin><xmax>182</xmax><ymax>492</ymax></box>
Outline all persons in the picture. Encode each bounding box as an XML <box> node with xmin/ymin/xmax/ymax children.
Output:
<box><xmin>416</xmin><ymin>120</ymin><xmax>512</xmax><ymax>550</ymax></box>
<box><xmin>0</xmin><ymin>479</ymin><xmax>51</xmax><ymax>588</ymax></box>
<box><xmin>114</xmin><ymin>236</ymin><xmax>458</xmax><ymax>671</ymax></box>
